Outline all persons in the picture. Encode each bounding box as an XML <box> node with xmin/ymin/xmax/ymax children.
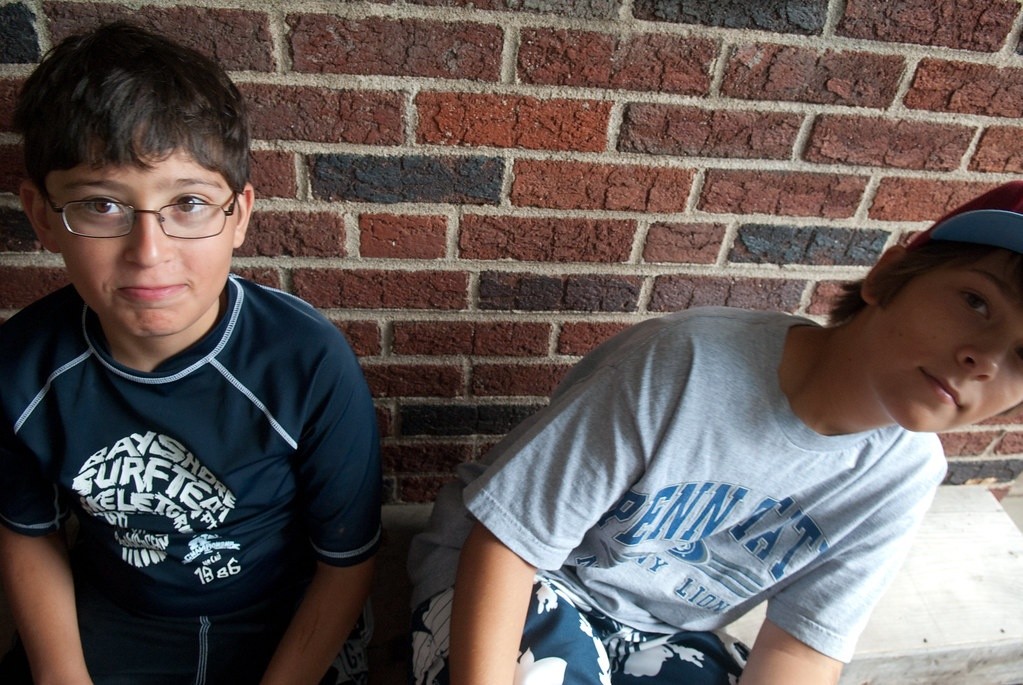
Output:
<box><xmin>400</xmin><ymin>180</ymin><xmax>1023</xmax><ymax>685</ymax></box>
<box><xmin>1</xmin><ymin>22</ymin><xmax>386</xmax><ymax>685</ymax></box>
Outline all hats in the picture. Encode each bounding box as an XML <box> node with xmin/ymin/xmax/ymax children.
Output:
<box><xmin>901</xmin><ymin>181</ymin><xmax>1023</xmax><ymax>275</ymax></box>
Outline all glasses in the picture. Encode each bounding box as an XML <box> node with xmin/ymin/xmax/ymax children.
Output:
<box><xmin>36</xmin><ymin>171</ymin><xmax>238</xmax><ymax>239</ymax></box>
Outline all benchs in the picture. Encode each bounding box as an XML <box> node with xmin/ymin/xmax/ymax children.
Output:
<box><xmin>387</xmin><ymin>482</ymin><xmax>1023</xmax><ymax>685</ymax></box>
